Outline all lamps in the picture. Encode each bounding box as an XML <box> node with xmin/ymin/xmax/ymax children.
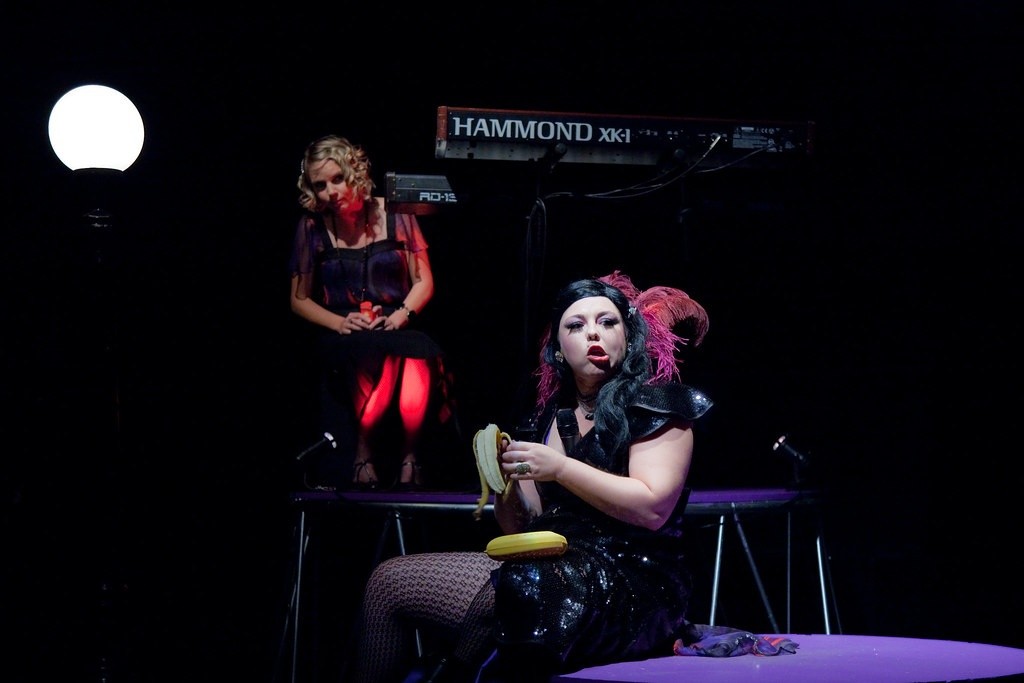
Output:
<box><xmin>771</xmin><ymin>433</ymin><xmax>813</xmax><ymax>489</ymax></box>
<box><xmin>295</xmin><ymin>431</ymin><xmax>338</xmax><ymax>491</ymax></box>
<box><xmin>48</xmin><ymin>83</ymin><xmax>144</xmax><ymax>683</ymax></box>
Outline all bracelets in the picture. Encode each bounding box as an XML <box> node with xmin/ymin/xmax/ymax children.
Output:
<box><xmin>395</xmin><ymin>303</ymin><xmax>417</xmax><ymax>320</ymax></box>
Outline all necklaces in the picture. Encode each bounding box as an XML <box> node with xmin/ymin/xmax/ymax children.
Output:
<box><xmin>577</xmin><ymin>390</ymin><xmax>598</xmax><ymax>420</ymax></box>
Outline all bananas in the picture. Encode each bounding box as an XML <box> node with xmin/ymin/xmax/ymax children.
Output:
<box><xmin>472</xmin><ymin>424</ymin><xmax>515</xmax><ymax>517</ymax></box>
<box><xmin>484</xmin><ymin>531</ymin><xmax>569</xmax><ymax>561</ymax></box>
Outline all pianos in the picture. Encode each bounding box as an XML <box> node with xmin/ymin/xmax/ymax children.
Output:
<box><xmin>435</xmin><ymin>104</ymin><xmax>817</xmax><ymax>164</ymax></box>
<box><xmin>385</xmin><ymin>171</ymin><xmax>511</xmax><ymax>214</ymax></box>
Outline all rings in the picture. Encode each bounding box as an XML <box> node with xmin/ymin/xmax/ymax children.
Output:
<box><xmin>515</xmin><ymin>462</ymin><xmax>530</xmax><ymax>474</ymax></box>
<box><xmin>351</xmin><ymin>319</ymin><xmax>353</xmax><ymax>323</ymax></box>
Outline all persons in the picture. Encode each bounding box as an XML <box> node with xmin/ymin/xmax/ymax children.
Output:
<box><xmin>286</xmin><ymin>134</ymin><xmax>452</xmax><ymax>494</ymax></box>
<box><xmin>350</xmin><ymin>269</ymin><xmax>715</xmax><ymax>683</ymax></box>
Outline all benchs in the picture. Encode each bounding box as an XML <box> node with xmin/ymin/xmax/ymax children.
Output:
<box><xmin>476</xmin><ymin>628</ymin><xmax>1024</xmax><ymax>683</ymax></box>
